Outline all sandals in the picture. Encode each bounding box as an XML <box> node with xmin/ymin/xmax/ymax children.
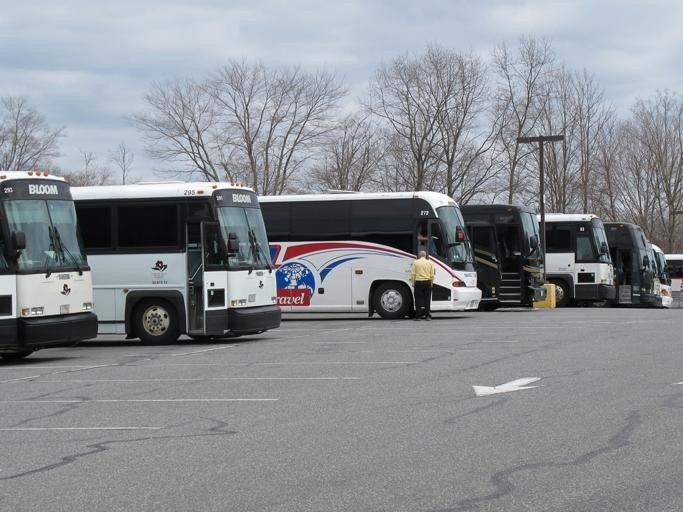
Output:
<box><xmin>69</xmin><ymin>181</ymin><xmax>283</xmax><ymax>347</ymax></box>
<box><xmin>1</xmin><ymin>171</ymin><xmax>98</xmax><ymax>361</ymax></box>
<box><xmin>458</xmin><ymin>204</ymin><xmax>683</xmax><ymax>312</ymax></box>
<box><xmin>218</xmin><ymin>190</ymin><xmax>484</xmax><ymax>320</ymax></box>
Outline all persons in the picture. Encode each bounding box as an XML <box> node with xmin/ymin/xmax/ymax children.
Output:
<box><xmin>409</xmin><ymin>250</ymin><xmax>435</xmax><ymax>321</ymax></box>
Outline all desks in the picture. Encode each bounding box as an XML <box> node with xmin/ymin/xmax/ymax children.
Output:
<box><xmin>516</xmin><ymin>134</ymin><xmax>566</xmax><ymax>286</ymax></box>
